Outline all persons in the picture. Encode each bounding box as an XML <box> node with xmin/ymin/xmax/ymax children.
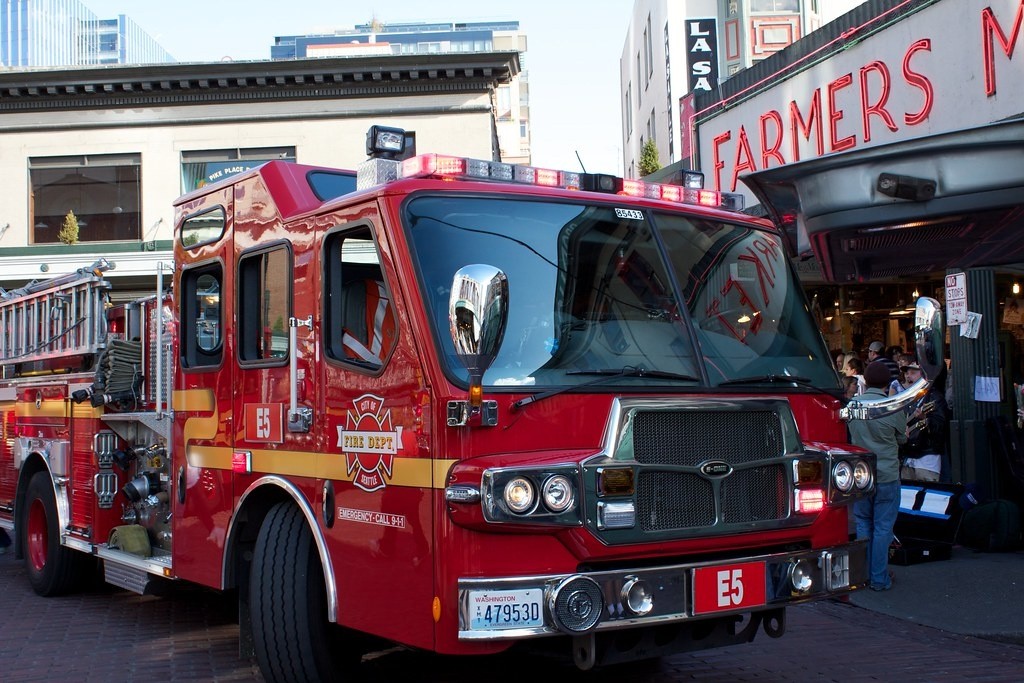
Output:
<box><xmin>846</xmin><ymin>362</ymin><xmax>906</xmax><ymax>590</ymax></box>
<box><xmin>829</xmin><ymin>341</ymin><xmax>952</xmax><ymax>482</ymax></box>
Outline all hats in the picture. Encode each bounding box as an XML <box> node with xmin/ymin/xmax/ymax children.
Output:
<box><xmin>899</xmin><ymin>360</ymin><xmax>921</xmax><ymax>370</ymax></box>
<box><xmin>863</xmin><ymin>362</ymin><xmax>892</xmax><ymax>387</ymax></box>
<box><xmin>869</xmin><ymin>341</ymin><xmax>885</xmax><ymax>352</ymax></box>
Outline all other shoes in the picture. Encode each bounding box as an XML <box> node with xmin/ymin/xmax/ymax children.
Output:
<box><xmin>870</xmin><ymin>579</ymin><xmax>893</xmax><ymax>591</ymax></box>
<box><xmin>889</xmin><ymin>569</ymin><xmax>896</xmax><ymax>579</ymax></box>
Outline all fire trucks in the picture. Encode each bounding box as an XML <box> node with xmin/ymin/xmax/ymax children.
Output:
<box><xmin>0</xmin><ymin>124</ymin><xmax>943</xmax><ymax>683</ymax></box>
